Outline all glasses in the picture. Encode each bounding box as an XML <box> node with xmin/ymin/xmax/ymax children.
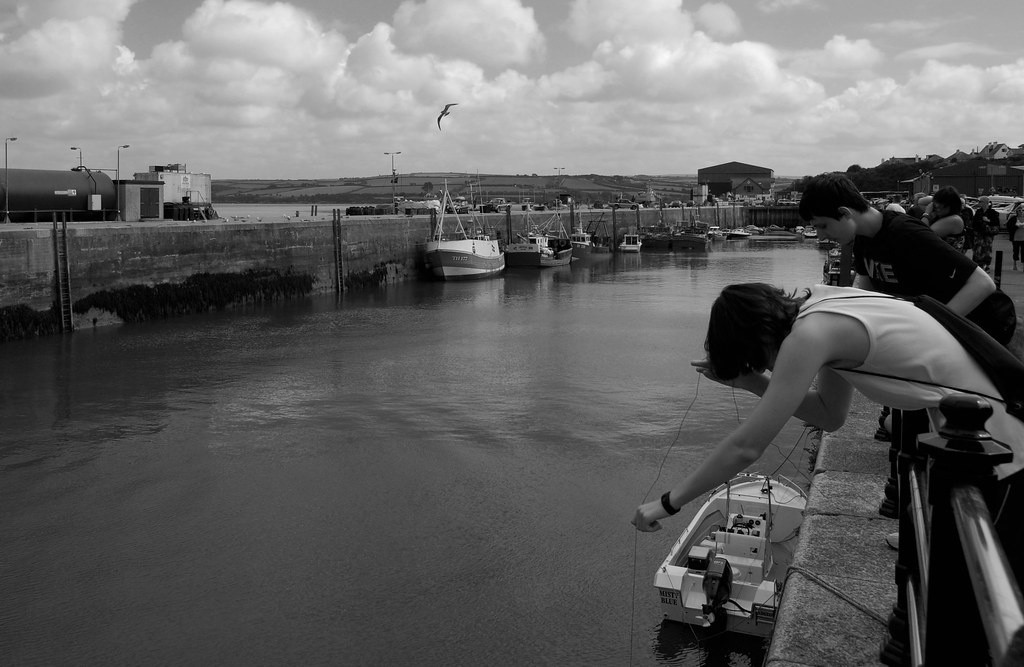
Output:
<box><xmin>979</xmin><ymin>201</ymin><xmax>983</xmax><ymax>203</ymax></box>
<box><xmin>1017</xmin><ymin>209</ymin><xmax>1024</xmax><ymax>212</ymax></box>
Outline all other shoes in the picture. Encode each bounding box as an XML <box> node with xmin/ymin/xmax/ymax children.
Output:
<box><xmin>886</xmin><ymin>531</ymin><xmax>899</xmax><ymax>550</ymax></box>
<box><xmin>984</xmin><ymin>266</ymin><xmax>991</xmax><ymax>274</ymax></box>
<box><xmin>1013</xmin><ymin>266</ymin><xmax>1017</xmax><ymax>270</ymax></box>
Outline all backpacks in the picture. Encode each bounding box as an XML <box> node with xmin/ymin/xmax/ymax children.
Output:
<box><xmin>795</xmin><ymin>289</ymin><xmax>1024</xmax><ymax>424</ymax></box>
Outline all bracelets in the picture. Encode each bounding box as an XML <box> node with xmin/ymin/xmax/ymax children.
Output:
<box><xmin>922</xmin><ymin>212</ymin><xmax>931</xmax><ymax>219</ymax></box>
<box><xmin>659</xmin><ymin>491</ymin><xmax>682</xmax><ymax>517</ymax></box>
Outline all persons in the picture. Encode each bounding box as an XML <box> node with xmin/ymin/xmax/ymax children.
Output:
<box><xmin>883</xmin><ymin>185</ymin><xmax>1024</xmax><ymax>274</ymax></box>
<box><xmin>629</xmin><ymin>282</ymin><xmax>1024</xmax><ymax>667</ymax></box>
<box><xmin>797</xmin><ymin>174</ymin><xmax>1024</xmax><ymax>551</ymax></box>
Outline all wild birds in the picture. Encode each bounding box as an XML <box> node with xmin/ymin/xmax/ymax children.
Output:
<box><xmin>437</xmin><ymin>103</ymin><xmax>459</xmax><ymax>131</ymax></box>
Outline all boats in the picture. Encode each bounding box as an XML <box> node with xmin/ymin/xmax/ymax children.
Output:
<box><xmin>424</xmin><ymin>178</ymin><xmax>505</xmax><ymax>282</ymax></box>
<box><xmin>617</xmin><ymin>233</ymin><xmax>643</xmax><ymax>253</ymax></box>
<box><xmin>501</xmin><ymin>199</ymin><xmax>574</xmax><ymax>269</ymax></box>
<box><xmin>571</xmin><ymin>203</ymin><xmax>834</xmax><ymax>254</ymax></box>
<box><xmin>653</xmin><ymin>471</ymin><xmax>809</xmax><ymax>638</ymax></box>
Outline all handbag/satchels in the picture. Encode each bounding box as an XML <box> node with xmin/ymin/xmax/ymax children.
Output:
<box><xmin>964</xmin><ymin>288</ymin><xmax>1016</xmax><ymax>346</ymax></box>
<box><xmin>985</xmin><ymin>224</ymin><xmax>1000</xmax><ymax>234</ymax></box>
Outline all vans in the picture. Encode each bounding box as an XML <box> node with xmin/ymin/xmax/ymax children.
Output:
<box><xmin>668</xmin><ymin>200</ymin><xmax>680</xmax><ymax>208</ymax></box>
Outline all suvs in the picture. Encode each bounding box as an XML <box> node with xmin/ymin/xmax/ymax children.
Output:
<box><xmin>447</xmin><ymin>196</ymin><xmax>469</xmax><ymax>213</ymax></box>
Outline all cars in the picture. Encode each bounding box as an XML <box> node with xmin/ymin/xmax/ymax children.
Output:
<box><xmin>965</xmin><ymin>193</ymin><xmax>1024</xmax><ymax>231</ymax></box>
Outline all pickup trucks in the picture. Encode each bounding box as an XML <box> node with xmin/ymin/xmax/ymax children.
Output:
<box><xmin>608</xmin><ymin>199</ymin><xmax>637</xmax><ymax>210</ymax></box>
<box><xmin>486</xmin><ymin>198</ymin><xmax>512</xmax><ymax>213</ymax></box>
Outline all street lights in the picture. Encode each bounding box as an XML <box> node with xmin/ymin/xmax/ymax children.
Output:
<box><xmin>71</xmin><ymin>147</ymin><xmax>82</xmax><ymax>171</ymax></box>
<box><xmin>553</xmin><ymin>167</ymin><xmax>565</xmax><ymax>200</ymax></box>
<box><xmin>2</xmin><ymin>137</ymin><xmax>17</xmax><ymax>223</ymax></box>
<box><xmin>115</xmin><ymin>145</ymin><xmax>130</xmax><ymax>221</ymax></box>
<box><xmin>384</xmin><ymin>152</ymin><xmax>401</xmax><ymax>201</ymax></box>
